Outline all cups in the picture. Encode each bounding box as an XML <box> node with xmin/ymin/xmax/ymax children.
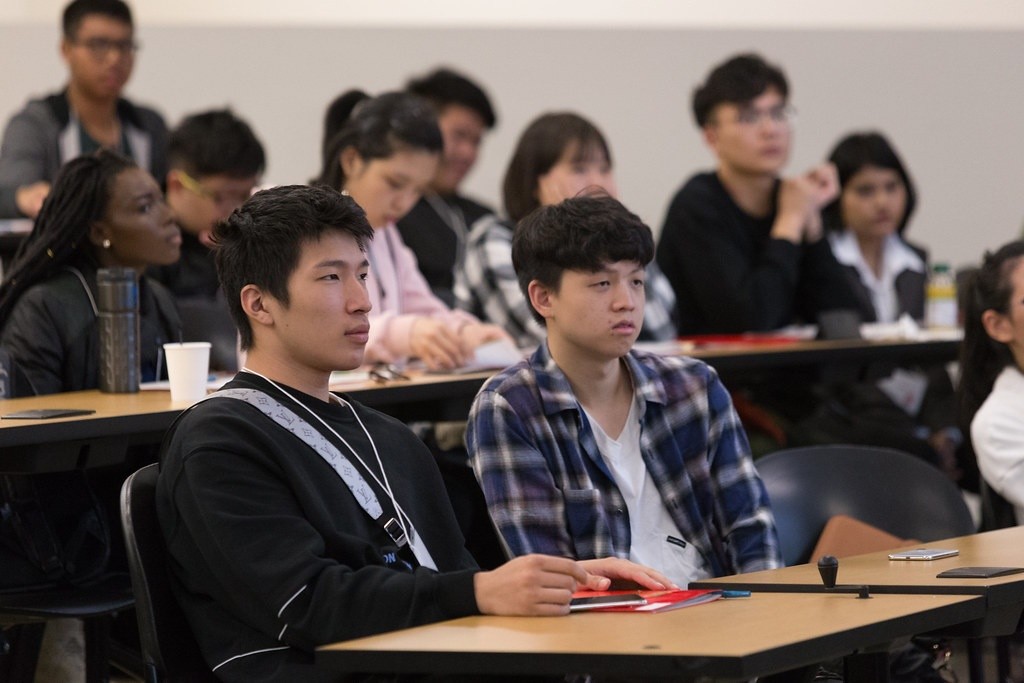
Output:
<box><xmin>163</xmin><ymin>342</ymin><xmax>210</xmax><ymax>403</ymax></box>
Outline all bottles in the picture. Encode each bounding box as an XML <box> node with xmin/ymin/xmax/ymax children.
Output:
<box><xmin>923</xmin><ymin>264</ymin><xmax>959</xmax><ymax>326</ymax></box>
<box><xmin>96</xmin><ymin>268</ymin><xmax>141</xmax><ymax>394</ymax></box>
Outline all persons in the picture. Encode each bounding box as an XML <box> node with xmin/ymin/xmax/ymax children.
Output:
<box><xmin>150</xmin><ymin>185</ymin><xmax>680</xmax><ymax>683</ymax></box>
<box><xmin>395</xmin><ymin>68</ymin><xmax>498</xmax><ymax>303</ymax></box>
<box><xmin>825</xmin><ymin>133</ymin><xmax>959</xmax><ymax>480</ymax></box>
<box><xmin>306</xmin><ymin>89</ymin><xmax>516</xmax><ymax>375</ymax></box>
<box><xmin>466</xmin><ymin>195</ymin><xmax>780</xmax><ymax>683</ymax></box>
<box><xmin>456</xmin><ymin>112</ymin><xmax>678</xmax><ymax>343</ymax></box>
<box><xmin>655</xmin><ymin>54</ymin><xmax>840</xmax><ymax>446</ymax></box>
<box><xmin>1</xmin><ymin>150</ymin><xmax>183</xmax><ymax>392</ymax></box>
<box><xmin>152</xmin><ymin>110</ymin><xmax>268</xmax><ymax>377</ymax></box>
<box><xmin>961</xmin><ymin>239</ymin><xmax>1024</xmax><ymax>529</ymax></box>
<box><xmin>1</xmin><ymin>0</ymin><xmax>170</xmax><ymax>218</ymax></box>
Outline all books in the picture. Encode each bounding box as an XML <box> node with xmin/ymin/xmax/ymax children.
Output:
<box><xmin>576</xmin><ymin>590</ymin><xmax>723</xmax><ymax>613</ymax></box>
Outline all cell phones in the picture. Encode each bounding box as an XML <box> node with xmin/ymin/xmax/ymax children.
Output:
<box><xmin>888</xmin><ymin>549</ymin><xmax>960</xmax><ymax>561</ymax></box>
<box><xmin>569</xmin><ymin>594</ymin><xmax>647</xmax><ymax>611</ymax></box>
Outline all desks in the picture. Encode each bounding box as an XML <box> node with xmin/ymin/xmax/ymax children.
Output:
<box><xmin>688</xmin><ymin>524</ymin><xmax>1024</xmax><ymax>683</ymax></box>
<box><xmin>0</xmin><ymin>322</ymin><xmax>964</xmax><ymax>683</ymax></box>
<box><xmin>313</xmin><ymin>589</ymin><xmax>988</xmax><ymax>683</ymax></box>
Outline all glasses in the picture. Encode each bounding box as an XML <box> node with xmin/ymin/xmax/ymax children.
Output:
<box><xmin>714</xmin><ymin>104</ymin><xmax>797</xmax><ymax>125</ymax></box>
<box><xmin>71</xmin><ymin>37</ymin><xmax>137</xmax><ymax>56</ymax></box>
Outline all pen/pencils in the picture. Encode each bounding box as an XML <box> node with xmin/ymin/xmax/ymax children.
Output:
<box><xmin>712</xmin><ymin>590</ymin><xmax>751</xmax><ymax>597</ymax></box>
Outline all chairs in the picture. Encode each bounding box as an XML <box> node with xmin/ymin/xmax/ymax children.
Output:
<box><xmin>0</xmin><ymin>585</ymin><xmax>136</xmax><ymax>683</ymax></box>
<box><xmin>752</xmin><ymin>444</ymin><xmax>974</xmax><ymax>569</ymax></box>
<box><xmin>120</xmin><ymin>462</ymin><xmax>220</xmax><ymax>683</ymax></box>
